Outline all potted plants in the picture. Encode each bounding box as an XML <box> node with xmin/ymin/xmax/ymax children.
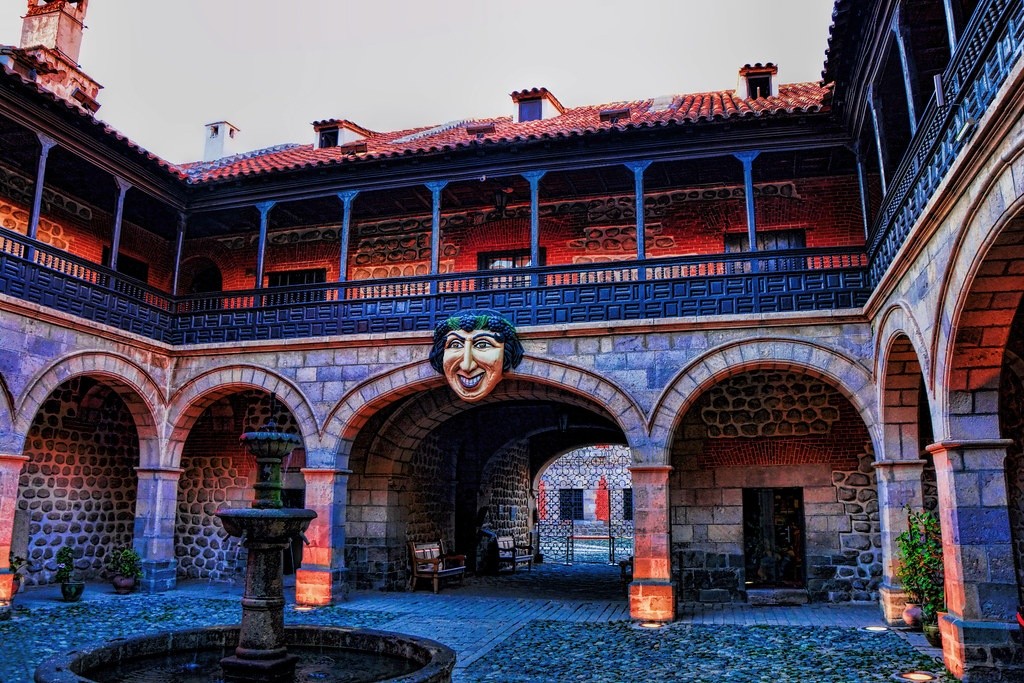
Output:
<box><xmin>104</xmin><ymin>549</ymin><xmax>143</xmax><ymax>595</ymax></box>
<box><xmin>889</xmin><ymin>518</ymin><xmax>932</xmax><ymax>631</ymax></box>
<box><xmin>55</xmin><ymin>547</ymin><xmax>85</xmax><ymax>602</ymax></box>
<box><xmin>8</xmin><ymin>551</ymin><xmax>34</xmax><ymax>602</ymax></box>
<box><xmin>900</xmin><ymin>501</ymin><xmax>947</xmax><ymax>647</ymax></box>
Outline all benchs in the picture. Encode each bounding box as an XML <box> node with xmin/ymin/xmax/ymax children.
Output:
<box><xmin>494</xmin><ymin>533</ymin><xmax>534</xmax><ymax>574</ymax></box>
<box><xmin>406</xmin><ymin>539</ymin><xmax>466</xmax><ymax>595</ymax></box>
